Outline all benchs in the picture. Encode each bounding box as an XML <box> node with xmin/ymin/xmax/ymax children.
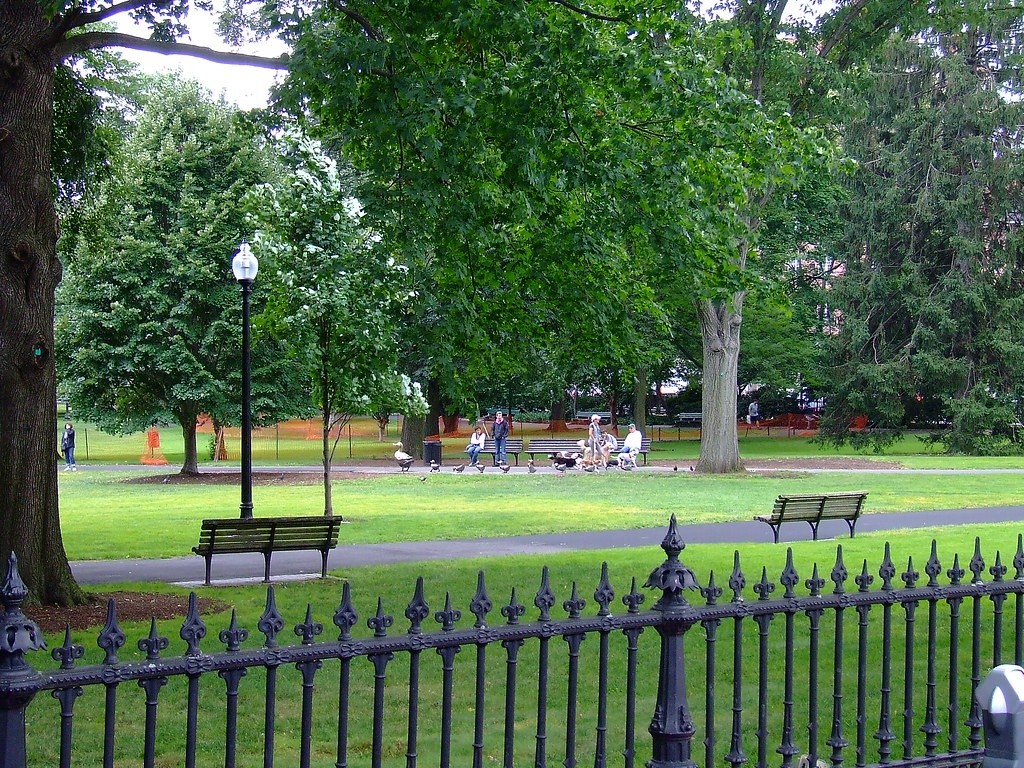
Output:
<box><xmin>675</xmin><ymin>413</ymin><xmax>703</xmax><ymax>422</ymax></box>
<box><xmin>194</xmin><ymin>516</ymin><xmax>342</xmax><ymax>583</ymax></box>
<box><xmin>755</xmin><ymin>490</ymin><xmax>869</xmax><ymax>544</ymax></box>
<box><xmin>467</xmin><ymin>441</ymin><xmax>521</xmax><ymax>468</ymax></box>
<box><xmin>526</xmin><ymin>437</ymin><xmax>652</xmax><ymax>467</ymax></box>
<box><xmin>573</xmin><ymin>411</ymin><xmax>612</xmax><ymax>425</ymax></box>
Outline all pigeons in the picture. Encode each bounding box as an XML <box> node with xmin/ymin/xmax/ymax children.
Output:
<box><xmin>420</xmin><ymin>477</ymin><xmax>427</xmax><ymax>483</ymax></box>
<box><xmin>278</xmin><ymin>473</ymin><xmax>285</xmax><ymax>481</ymax></box>
<box><xmin>674</xmin><ymin>466</ymin><xmax>677</xmax><ymax>471</ymax></box>
<box><xmin>690</xmin><ymin>465</ymin><xmax>693</xmax><ymax>471</ymax></box>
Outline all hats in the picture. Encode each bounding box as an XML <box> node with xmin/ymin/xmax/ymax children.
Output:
<box><xmin>628</xmin><ymin>423</ymin><xmax>635</xmax><ymax>428</ymax></box>
<box><xmin>497</xmin><ymin>411</ymin><xmax>503</xmax><ymax>417</ymax></box>
<box><xmin>591</xmin><ymin>414</ymin><xmax>601</xmax><ymax>422</ymax></box>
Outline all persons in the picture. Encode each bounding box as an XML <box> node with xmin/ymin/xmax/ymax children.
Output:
<box><xmin>589</xmin><ymin>414</ymin><xmax>612</xmax><ymax>468</ymax></box>
<box><xmin>601</xmin><ymin>431</ymin><xmax>618</xmax><ymax>451</ymax></box>
<box><xmin>748</xmin><ymin>400</ymin><xmax>762</xmax><ymax>430</ymax></box>
<box><xmin>467</xmin><ymin>427</ymin><xmax>486</xmax><ymax>467</ymax></box>
<box><xmin>61</xmin><ymin>423</ymin><xmax>76</xmax><ymax>472</ymax></box>
<box><xmin>492</xmin><ymin>411</ymin><xmax>508</xmax><ymax>465</ymax></box>
<box><xmin>623</xmin><ymin>424</ymin><xmax>642</xmax><ymax>452</ymax></box>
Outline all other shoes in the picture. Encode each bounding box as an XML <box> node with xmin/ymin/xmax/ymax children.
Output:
<box><xmin>757</xmin><ymin>427</ymin><xmax>760</xmax><ymax>428</ymax></box>
<box><xmin>748</xmin><ymin>427</ymin><xmax>752</xmax><ymax>429</ymax></box>
<box><xmin>469</xmin><ymin>463</ymin><xmax>473</xmax><ymax>467</ymax></box>
<box><xmin>473</xmin><ymin>463</ymin><xmax>477</xmax><ymax>465</ymax></box>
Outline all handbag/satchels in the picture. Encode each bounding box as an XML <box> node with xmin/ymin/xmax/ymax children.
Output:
<box><xmin>466</xmin><ymin>433</ymin><xmax>477</xmax><ymax>450</ymax></box>
<box><xmin>747</xmin><ymin>415</ymin><xmax>751</xmax><ymax>424</ymax></box>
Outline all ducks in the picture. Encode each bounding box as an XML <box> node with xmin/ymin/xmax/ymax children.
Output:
<box><xmin>475</xmin><ymin>461</ymin><xmax>485</xmax><ymax>473</ymax></box>
<box><xmin>430</xmin><ymin>460</ymin><xmax>440</xmax><ymax>472</ymax></box>
<box><xmin>453</xmin><ymin>464</ymin><xmax>465</xmax><ymax>473</ymax></box>
<box><xmin>497</xmin><ymin>461</ymin><xmax>510</xmax><ymax>473</ymax></box>
<box><xmin>555</xmin><ymin>463</ymin><xmax>566</xmax><ymax>473</ymax></box>
<box><xmin>528</xmin><ymin>460</ymin><xmax>536</xmax><ymax>473</ymax></box>
<box><xmin>582</xmin><ymin>465</ymin><xmax>595</xmax><ymax>472</ymax></box>
<box><xmin>393</xmin><ymin>442</ymin><xmax>414</xmax><ymax>471</ymax></box>
<box><xmin>620</xmin><ymin>462</ymin><xmax>633</xmax><ymax>471</ymax></box>
